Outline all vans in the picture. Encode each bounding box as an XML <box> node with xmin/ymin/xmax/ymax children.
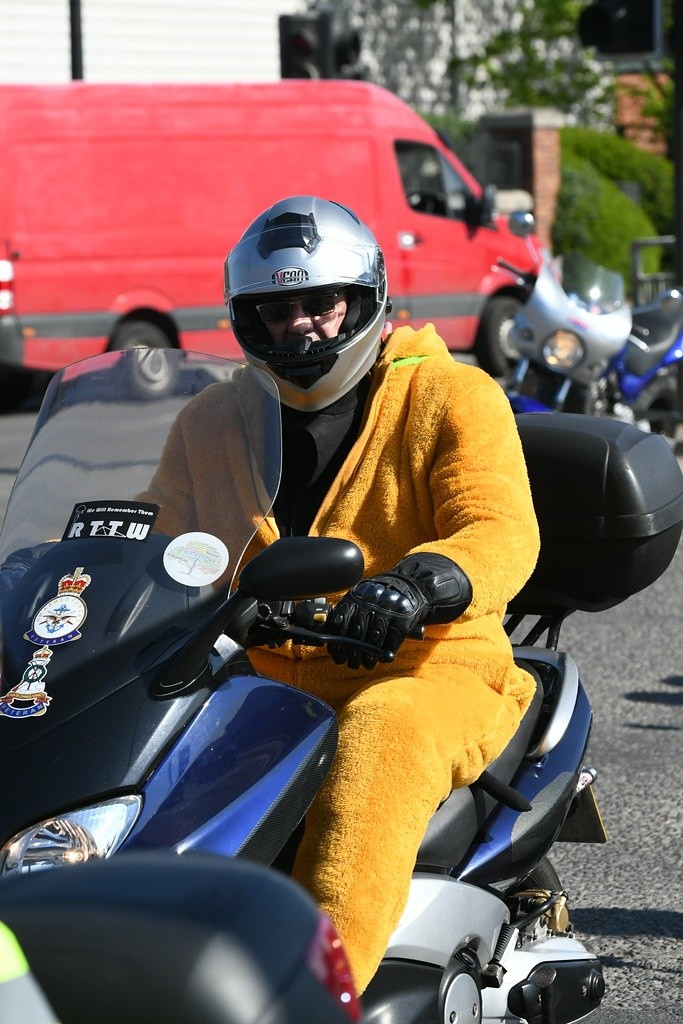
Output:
<box><xmin>2</xmin><ymin>80</ymin><xmax>539</xmax><ymax>403</ymax></box>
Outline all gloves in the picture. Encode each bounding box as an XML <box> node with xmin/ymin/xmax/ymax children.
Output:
<box><xmin>327</xmin><ymin>552</ymin><xmax>472</xmax><ymax>669</ymax></box>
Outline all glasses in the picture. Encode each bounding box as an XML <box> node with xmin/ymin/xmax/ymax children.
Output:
<box><xmin>255</xmin><ymin>290</ymin><xmax>338</xmax><ymax>323</ymax></box>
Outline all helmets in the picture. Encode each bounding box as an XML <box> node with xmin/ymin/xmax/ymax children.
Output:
<box><xmin>224</xmin><ymin>197</ymin><xmax>391</xmax><ymax>412</ymax></box>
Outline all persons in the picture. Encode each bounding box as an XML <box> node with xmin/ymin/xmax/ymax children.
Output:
<box><xmin>97</xmin><ymin>193</ymin><xmax>544</xmax><ymax>1001</ymax></box>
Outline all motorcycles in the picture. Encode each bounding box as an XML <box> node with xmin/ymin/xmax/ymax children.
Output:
<box><xmin>0</xmin><ymin>341</ymin><xmax>681</xmax><ymax>1024</ymax></box>
<box><xmin>482</xmin><ymin>207</ymin><xmax>681</xmax><ymax>478</ymax></box>
